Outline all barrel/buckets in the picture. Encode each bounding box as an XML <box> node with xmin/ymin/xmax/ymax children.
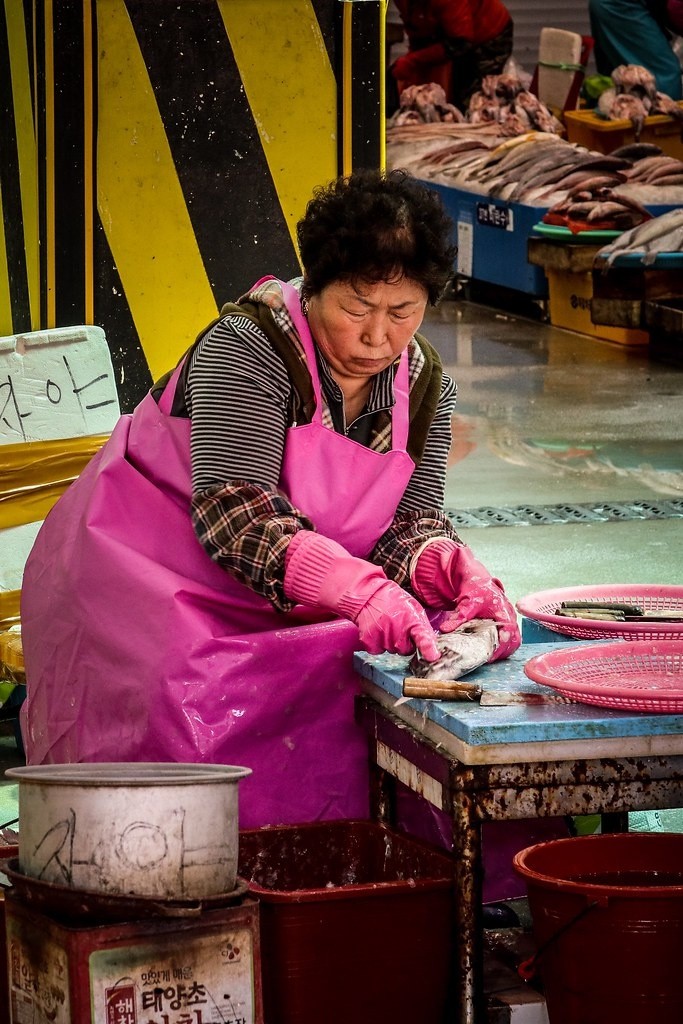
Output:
<box><xmin>513</xmin><ymin>832</ymin><xmax>683</xmax><ymax>1024</ymax></box>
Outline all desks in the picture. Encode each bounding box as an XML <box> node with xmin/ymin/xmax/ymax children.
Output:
<box><xmin>349</xmin><ymin>617</ymin><xmax>683</xmax><ymax>1024</ymax></box>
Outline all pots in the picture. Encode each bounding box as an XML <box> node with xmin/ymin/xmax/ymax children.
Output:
<box><xmin>5</xmin><ymin>763</ymin><xmax>252</xmax><ymax>899</ymax></box>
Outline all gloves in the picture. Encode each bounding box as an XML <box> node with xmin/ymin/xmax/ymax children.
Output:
<box><xmin>416</xmin><ymin>539</ymin><xmax>521</xmax><ymax>665</ymax></box>
<box><xmin>283</xmin><ymin>529</ymin><xmax>441</xmax><ymax>662</ymax></box>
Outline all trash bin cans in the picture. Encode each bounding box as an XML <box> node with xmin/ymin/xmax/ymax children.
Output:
<box><xmin>233</xmin><ymin>814</ymin><xmax>462</xmax><ymax>1024</ymax></box>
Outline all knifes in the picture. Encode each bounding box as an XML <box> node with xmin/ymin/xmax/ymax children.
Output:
<box><xmin>401</xmin><ymin>677</ymin><xmax>579</xmax><ymax>706</ymax></box>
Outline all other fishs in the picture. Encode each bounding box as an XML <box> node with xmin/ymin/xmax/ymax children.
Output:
<box><xmin>383</xmin><ymin>108</ymin><xmax>682</xmax><ymax>266</ymax></box>
<box><xmin>393</xmin><ymin>615</ymin><xmax>497</xmax><ymax>707</ymax></box>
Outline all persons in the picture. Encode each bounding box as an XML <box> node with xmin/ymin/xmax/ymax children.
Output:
<box><xmin>21</xmin><ymin>173</ymin><xmax>522</xmax><ymax>829</ymax></box>
<box><xmin>394</xmin><ymin>0</ymin><xmax>514</xmax><ymax>112</ymax></box>
<box><xmin>589</xmin><ymin>0</ymin><xmax>683</xmax><ymax>101</ymax></box>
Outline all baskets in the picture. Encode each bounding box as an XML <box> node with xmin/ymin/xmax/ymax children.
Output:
<box><xmin>523</xmin><ymin>640</ymin><xmax>683</xmax><ymax>713</ymax></box>
<box><xmin>516</xmin><ymin>584</ymin><xmax>683</xmax><ymax>641</ymax></box>
